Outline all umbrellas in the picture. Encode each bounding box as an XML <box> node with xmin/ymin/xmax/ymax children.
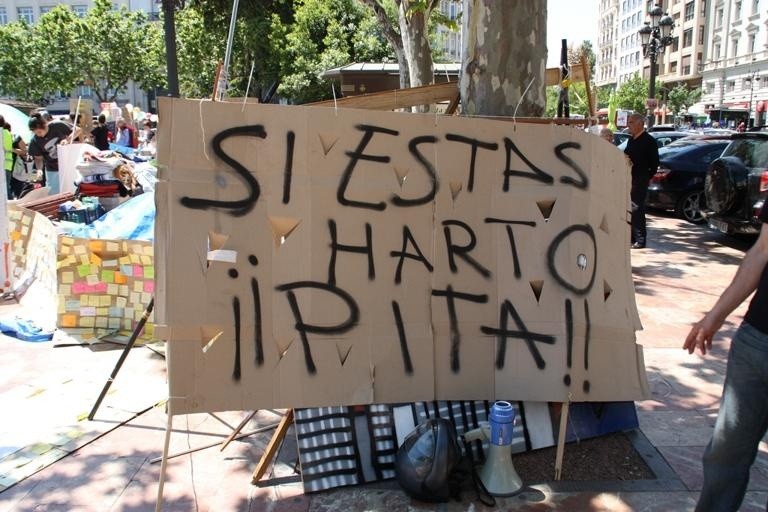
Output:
<box><xmin>608</xmin><ymin>89</ymin><xmax>619</xmax><ymax>130</ymax></box>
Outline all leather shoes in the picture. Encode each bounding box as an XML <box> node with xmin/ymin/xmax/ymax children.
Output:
<box><xmin>631</xmin><ymin>241</ymin><xmax>646</xmax><ymax>249</ymax></box>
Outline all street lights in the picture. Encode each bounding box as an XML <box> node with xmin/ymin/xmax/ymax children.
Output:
<box><xmin>745</xmin><ymin>68</ymin><xmax>763</xmax><ymax>131</ymax></box>
<box><xmin>639</xmin><ymin>4</ymin><xmax>675</xmax><ymax>132</ymax></box>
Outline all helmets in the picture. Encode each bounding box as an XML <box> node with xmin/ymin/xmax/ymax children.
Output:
<box><xmin>394</xmin><ymin>418</ymin><xmax>475</xmax><ymax>504</ymax></box>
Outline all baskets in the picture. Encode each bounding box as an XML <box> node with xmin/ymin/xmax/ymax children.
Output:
<box><xmin>57</xmin><ymin>201</ymin><xmax>99</xmax><ymax>225</ymax></box>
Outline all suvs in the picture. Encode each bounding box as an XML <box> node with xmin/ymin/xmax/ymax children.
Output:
<box><xmin>704</xmin><ymin>131</ymin><xmax>767</xmax><ymax>240</ymax></box>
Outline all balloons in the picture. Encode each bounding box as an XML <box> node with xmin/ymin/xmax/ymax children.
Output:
<box><xmin>110</xmin><ymin>103</ymin><xmax>117</xmax><ymax>109</ymax></box>
<box><xmin>125</xmin><ymin>103</ymin><xmax>133</xmax><ymax>113</ymax></box>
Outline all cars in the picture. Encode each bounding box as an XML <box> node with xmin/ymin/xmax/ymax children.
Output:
<box><xmin>617</xmin><ymin>130</ymin><xmax>703</xmax><ymax>157</ymax></box>
<box><xmin>647</xmin><ymin>139</ymin><xmax>732</xmax><ymax>221</ymax></box>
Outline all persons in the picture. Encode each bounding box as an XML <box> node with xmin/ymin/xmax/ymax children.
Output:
<box><xmin>690</xmin><ymin>119</ymin><xmax>747</xmax><ymax>133</ymax></box>
<box><xmin>599</xmin><ymin>129</ymin><xmax>613</xmax><ymax>143</ymax></box>
<box><xmin>683</xmin><ymin>190</ymin><xmax>768</xmax><ymax>512</ymax></box>
<box><xmin>0</xmin><ymin>108</ymin><xmax>157</xmax><ymax>203</ymax></box>
<box><xmin>588</xmin><ymin>118</ymin><xmax>600</xmax><ymax>137</ymax></box>
<box><xmin>622</xmin><ymin>113</ymin><xmax>662</xmax><ymax>249</ymax></box>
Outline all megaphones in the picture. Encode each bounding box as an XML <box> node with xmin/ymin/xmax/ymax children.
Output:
<box><xmin>457</xmin><ymin>399</ymin><xmax>525</xmax><ymax>497</ymax></box>
